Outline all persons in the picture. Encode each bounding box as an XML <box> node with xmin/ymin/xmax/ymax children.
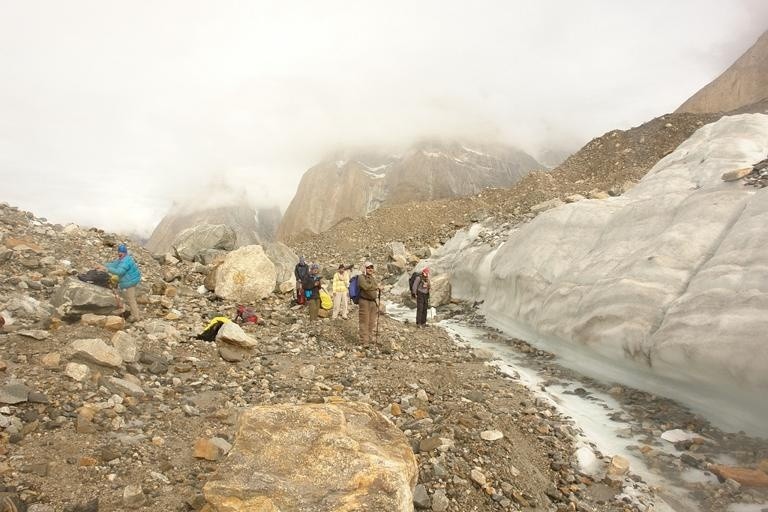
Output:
<box><xmin>97</xmin><ymin>244</ymin><xmax>141</xmax><ymax>321</ymax></box>
<box><xmin>411</xmin><ymin>267</ymin><xmax>431</xmax><ymax>329</ymax></box>
<box><xmin>293</xmin><ymin>256</ymin><xmax>322</xmax><ymax>320</ymax></box>
<box><xmin>331</xmin><ymin>264</ymin><xmax>350</xmax><ymax>321</ymax></box>
<box><xmin>356</xmin><ymin>261</ymin><xmax>384</xmax><ymax>348</ymax></box>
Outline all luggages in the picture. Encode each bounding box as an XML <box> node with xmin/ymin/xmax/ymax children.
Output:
<box><xmin>297</xmin><ymin>281</ymin><xmax>305</xmax><ymax>304</ymax></box>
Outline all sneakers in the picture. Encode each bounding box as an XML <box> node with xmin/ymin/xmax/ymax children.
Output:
<box><xmin>331</xmin><ymin>317</ymin><xmax>336</xmax><ymax>320</ymax></box>
<box><xmin>342</xmin><ymin>317</ymin><xmax>347</xmax><ymax>320</ymax></box>
<box><xmin>417</xmin><ymin>323</ymin><xmax>430</xmax><ymax>329</ymax></box>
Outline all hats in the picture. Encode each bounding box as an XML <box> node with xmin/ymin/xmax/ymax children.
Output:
<box><xmin>364</xmin><ymin>261</ymin><xmax>376</xmax><ymax>275</ymax></box>
<box><xmin>118</xmin><ymin>244</ymin><xmax>127</xmax><ymax>252</ymax></box>
<box><xmin>423</xmin><ymin>268</ymin><xmax>430</xmax><ymax>274</ymax></box>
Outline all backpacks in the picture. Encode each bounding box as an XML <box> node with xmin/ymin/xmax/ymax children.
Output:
<box><xmin>77</xmin><ymin>269</ymin><xmax>112</xmax><ymax>287</ymax></box>
<box><xmin>234</xmin><ymin>304</ymin><xmax>257</xmax><ymax>322</ymax></box>
<box><xmin>349</xmin><ymin>274</ymin><xmax>366</xmax><ymax>304</ymax></box>
<box><xmin>409</xmin><ymin>272</ymin><xmax>422</xmax><ymax>291</ymax></box>
